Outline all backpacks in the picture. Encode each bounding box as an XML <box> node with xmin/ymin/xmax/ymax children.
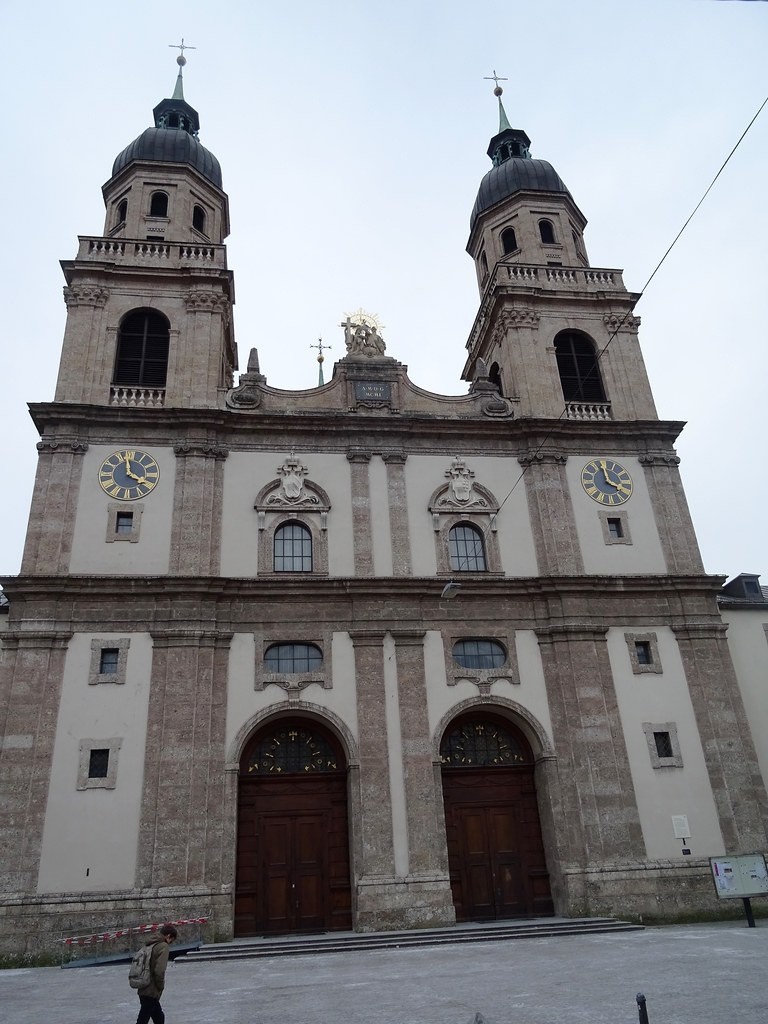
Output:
<box><xmin>129</xmin><ymin>941</ymin><xmax>159</xmax><ymax>988</ymax></box>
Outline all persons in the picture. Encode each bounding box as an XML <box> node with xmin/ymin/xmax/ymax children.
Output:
<box><xmin>136</xmin><ymin>924</ymin><xmax>177</xmax><ymax>1024</ymax></box>
<box><xmin>343</xmin><ymin>319</ymin><xmax>386</xmax><ymax>357</ymax></box>
